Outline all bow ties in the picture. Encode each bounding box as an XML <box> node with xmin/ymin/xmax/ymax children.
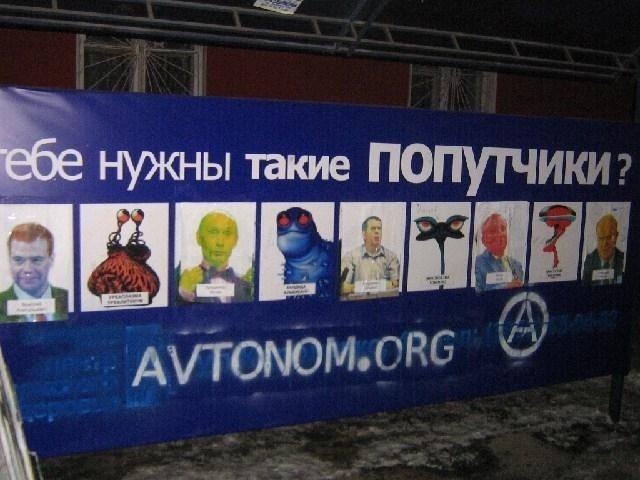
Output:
<box><xmin>208</xmin><ymin>267</ymin><xmax>233</xmax><ymax>281</ymax></box>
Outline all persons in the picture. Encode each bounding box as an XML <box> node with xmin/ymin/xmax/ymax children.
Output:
<box><xmin>341</xmin><ymin>216</ymin><xmax>399</xmax><ymax>300</ymax></box>
<box><xmin>582</xmin><ymin>213</ymin><xmax>624</xmax><ymax>283</ymax></box>
<box><xmin>178</xmin><ymin>210</ymin><xmax>252</xmax><ymax>304</ymax></box>
<box><xmin>0</xmin><ymin>223</ymin><xmax>69</xmax><ymax>323</ymax></box>
<box><xmin>475</xmin><ymin>212</ymin><xmax>523</xmax><ymax>292</ymax></box>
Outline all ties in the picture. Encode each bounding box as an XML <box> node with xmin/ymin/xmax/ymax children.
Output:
<box><xmin>34</xmin><ymin>298</ymin><xmax>46</xmax><ymax>322</ymax></box>
<box><xmin>601</xmin><ymin>262</ymin><xmax>611</xmax><ymax>285</ymax></box>
